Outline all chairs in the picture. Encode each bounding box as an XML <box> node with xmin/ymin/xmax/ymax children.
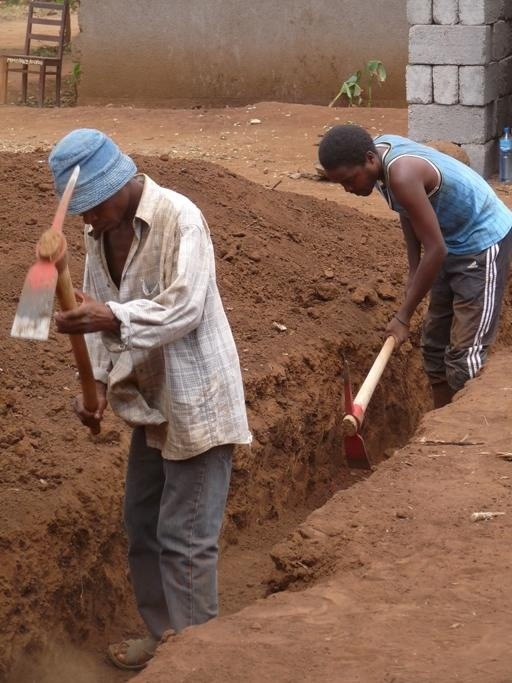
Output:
<box><xmin>3</xmin><ymin>0</ymin><xmax>70</xmax><ymax>107</ymax></box>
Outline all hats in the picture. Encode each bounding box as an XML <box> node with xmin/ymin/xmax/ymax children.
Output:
<box><xmin>47</xmin><ymin>127</ymin><xmax>138</xmax><ymax>216</ymax></box>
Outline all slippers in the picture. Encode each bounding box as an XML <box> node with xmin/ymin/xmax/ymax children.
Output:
<box><xmin>107</xmin><ymin>634</ymin><xmax>160</xmax><ymax>671</ymax></box>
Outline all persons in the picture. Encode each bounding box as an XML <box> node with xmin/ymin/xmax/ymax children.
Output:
<box><xmin>318</xmin><ymin>123</ymin><xmax>512</xmax><ymax>409</ymax></box>
<box><xmin>47</xmin><ymin>128</ymin><xmax>252</xmax><ymax>672</ymax></box>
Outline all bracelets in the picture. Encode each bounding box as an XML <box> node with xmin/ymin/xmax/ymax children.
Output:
<box><xmin>394</xmin><ymin>314</ymin><xmax>410</xmax><ymax>328</ymax></box>
